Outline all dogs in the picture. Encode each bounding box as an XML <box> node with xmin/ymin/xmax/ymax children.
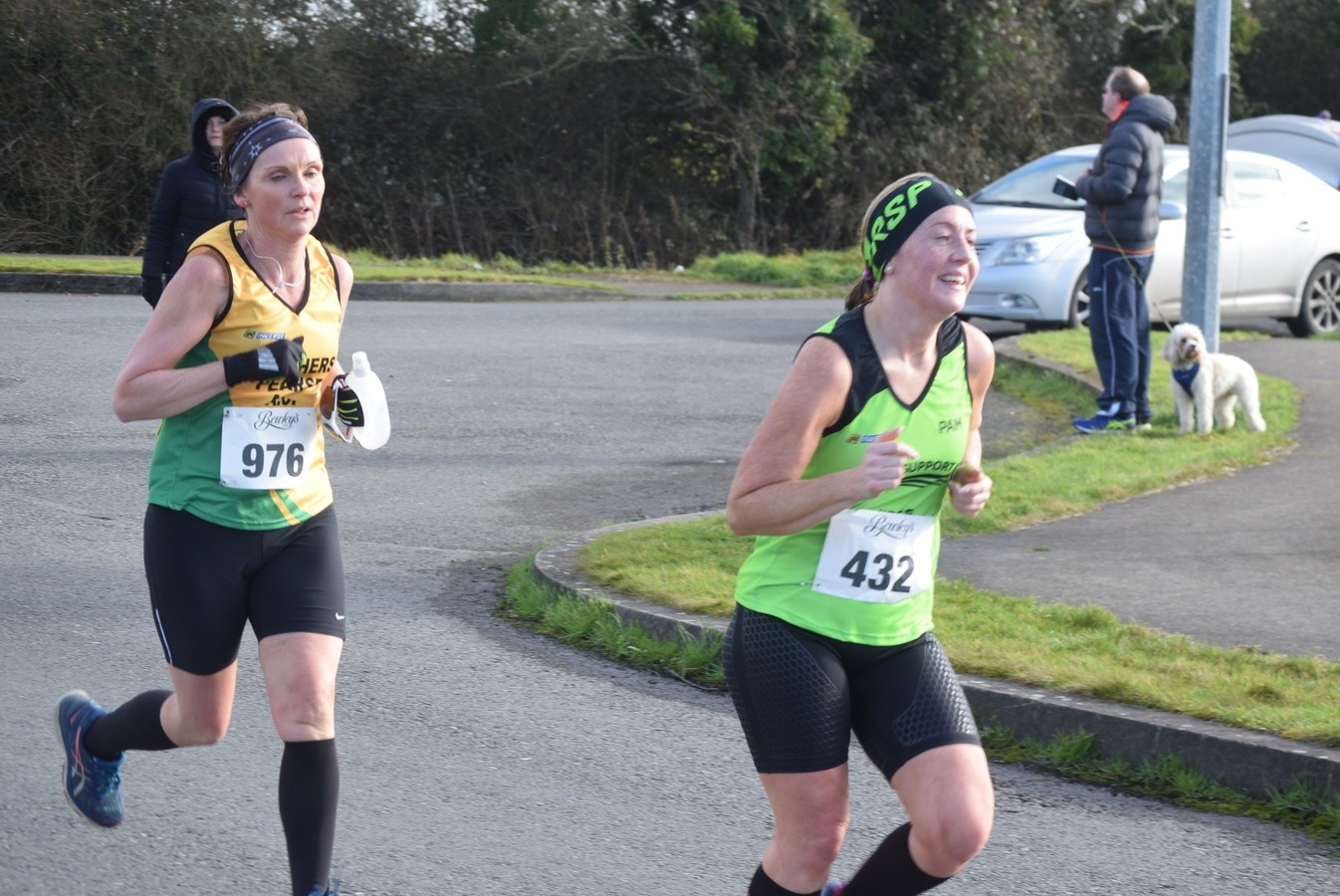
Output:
<box><xmin>1163</xmin><ymin>321</ymin><xmax>1268</xmax><ymax>436</ymax></box>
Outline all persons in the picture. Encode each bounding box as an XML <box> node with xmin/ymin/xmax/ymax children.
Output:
<box><xmin>54</xmin><ymin>100</ymin><xmax>360</xmax><ymax>896</ymax></box>
<box><xmin>1074</xmin><ymin>63</ymin><xmax>1176</xmax><ymax>436</ymax></box>
<box><xmin>718</xmin><ymin>165</ymin><xmax>999</xmax><ymax>893</ymax></box>
<box><xmin>139</xmin><ymin>96</ymin><xmax>244</xmax><ymax>309</ymax></box>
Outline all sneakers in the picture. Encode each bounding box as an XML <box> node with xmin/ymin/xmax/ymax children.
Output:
<box><xmin>1133</xmin><ymin>412</ymin><xmax>1152</xmax><ymax>430</ymax></box>
<box><xmin>52</xmin><ymin>688</ymin><xmax>126</xmax><ymax>828</ymax></box>
<box><xmin>1072</xmin><ymin>413</ymin><xmax>1136</xmax><ymax>435</ymax></box>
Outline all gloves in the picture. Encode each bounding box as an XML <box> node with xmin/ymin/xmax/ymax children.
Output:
<box><xmin>222</xmin><ymin>336</ymin><xmax>308</xmax><ymax>392</ymax></box>
<box><xmin>330</xmin><ymin>372</ymin><xmax>365</xmax><ymax>427</ymax></box>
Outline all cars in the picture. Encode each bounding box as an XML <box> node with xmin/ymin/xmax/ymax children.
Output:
<box><xmin>1226</xmin><ymin>113</ymin><xmax>1340</xmax><ymax>197</ymax></box>
<box><xmin>963</xmin><ymin>140</ymin><xmax>1340</xmax><ymax>338</ymax></box>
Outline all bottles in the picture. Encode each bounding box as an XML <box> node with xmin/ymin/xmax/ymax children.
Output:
<box><xmin>347</xmin><ymin>350</ymin><xmax>391</xmax><ymax>450</ymax></box>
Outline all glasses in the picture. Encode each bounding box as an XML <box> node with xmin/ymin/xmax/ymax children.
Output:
<box><xmin>319</xmin><ymin>379</ymin><xmax>355</xmax><ymax>445</ymax></box>
<box><xmin>1100</xmin><ymin>86</ymin><xmax>1114</xmax><ymax>95</ymax></box>
